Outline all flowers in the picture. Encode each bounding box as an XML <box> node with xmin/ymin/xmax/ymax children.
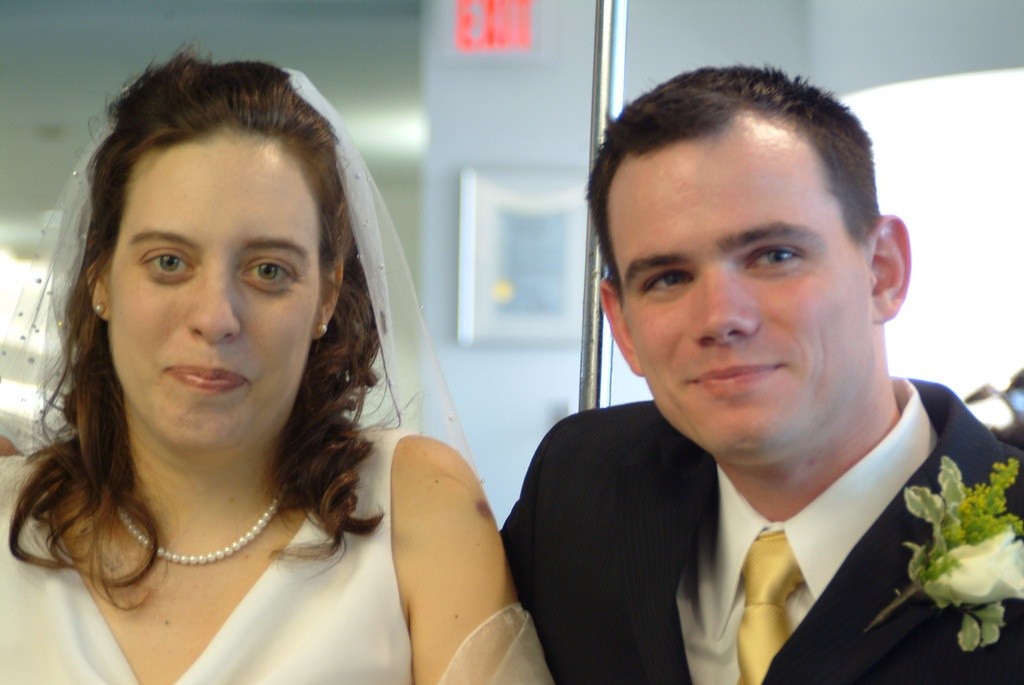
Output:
<box><xmin>864</xmin><ymin>456</ymin><xmax>1023</xmax><ymax>652</ymax></box>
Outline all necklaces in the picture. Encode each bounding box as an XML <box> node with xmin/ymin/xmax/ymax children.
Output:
<box><xmin>114</xmin><ymin>492</ymin><xmax>282</xmax><ymax>566</ymax></box>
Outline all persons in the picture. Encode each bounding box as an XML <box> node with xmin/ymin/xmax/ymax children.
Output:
<box><xmin>0</xmin><ymin>65</ymin><xmax>1024</xmax><ymax>685</ymax></box>
<box><xmin>0</xmin><ymin>41</ymin><xmax>552</xmax><ymax>685</ymax></box>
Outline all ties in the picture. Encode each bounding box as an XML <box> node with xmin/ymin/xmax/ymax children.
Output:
<box><xmin>737</xmin><ymin>530</ymin><xmax>805</xmax><ymax>685</ymax></box>
